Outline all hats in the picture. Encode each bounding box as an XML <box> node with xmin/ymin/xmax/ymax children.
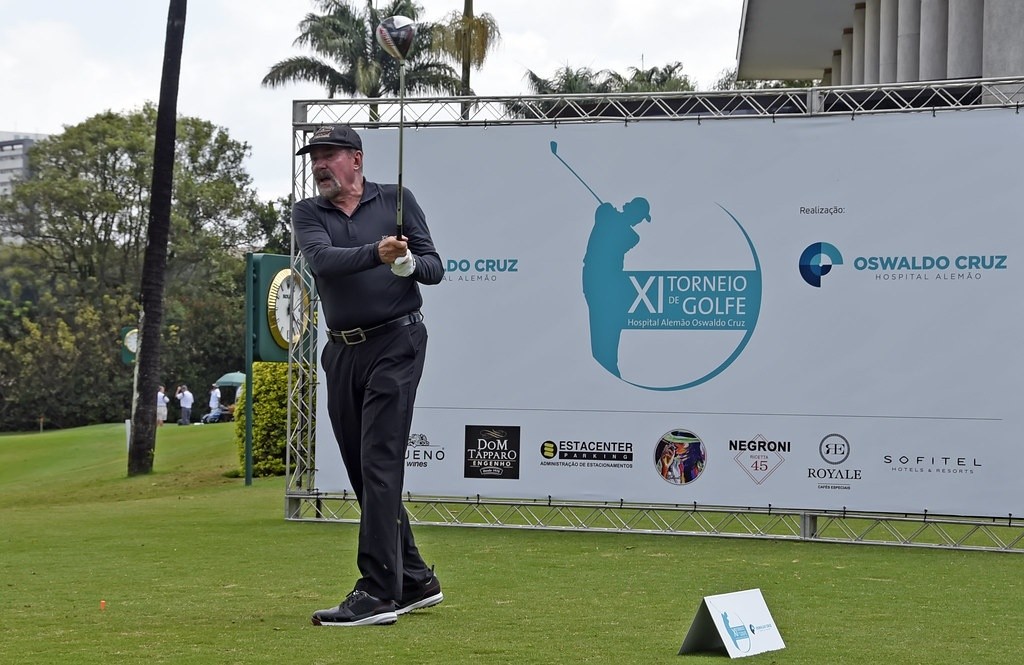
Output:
<box><xmin>212</xmin><ymin>383</ymin><xmax>219</xmax><ymax>387</ymax></box>
<box><xmin>296</xmin><ymin>125</ymin><xmax>361</xmax><ymax>155</ymax></box>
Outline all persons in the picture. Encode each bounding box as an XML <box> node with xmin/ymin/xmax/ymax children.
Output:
<box><xmin>175</xmin><ymin>384</ymin><xmax>193</xmax><ymax>425</ymax></box>
<box><xmin>208</xmin><ymin>383</ymin><xmax>221</xmax><ymax>423</ymax></box>
<box><xmin>156</xmin><ymin>386</ymin><xmax>171</xmax><ymax>427</ymax></box>
<box><xmin>291</xmin><ymin>124</ymin><xmax>445</xmax><ymax>625</ymax></box>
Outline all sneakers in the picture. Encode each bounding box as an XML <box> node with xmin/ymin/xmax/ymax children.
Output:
<box><xmin>311</xmin><ymin>589</ymin><xmax>398</xmax><ymax>626</ymax></box>
<box><xmin>396</xmin><ymin>565</ymin><xmax>443</xmax><ymax>615</ymax></box>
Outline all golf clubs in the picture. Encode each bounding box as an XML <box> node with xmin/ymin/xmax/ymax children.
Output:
<box><xmin>375</xmin><ymin>25</ymin><xmax>408</xmax><ymax>240</ymax></box>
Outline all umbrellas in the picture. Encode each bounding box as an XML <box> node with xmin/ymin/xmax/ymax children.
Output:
<box><xmin>212</xmin><ymin>371</ymin><xmax>249</xmax><ymax>387</ymax></box>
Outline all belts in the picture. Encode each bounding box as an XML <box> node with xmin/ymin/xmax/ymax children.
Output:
<box><xmin>325</xmin><ymin>313</ymin><xmax>420</xmax><ymax>346</ymax></box>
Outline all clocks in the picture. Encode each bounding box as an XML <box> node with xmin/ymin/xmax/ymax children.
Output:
<box><xmin>123</xmin><ymin>328</ymin><xmax>141</xmax><ymax>353</ymax></box>
<box><xmin>267</xmin><ymin>268</ymin><xmax>310</xmax><ymax>350</ymax></box>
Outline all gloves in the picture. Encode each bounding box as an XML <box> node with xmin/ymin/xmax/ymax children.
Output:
<box><xmin>391</xmin><ymin>248</ymin><xmax>416</xmax><ymax>279</ymax></box>
